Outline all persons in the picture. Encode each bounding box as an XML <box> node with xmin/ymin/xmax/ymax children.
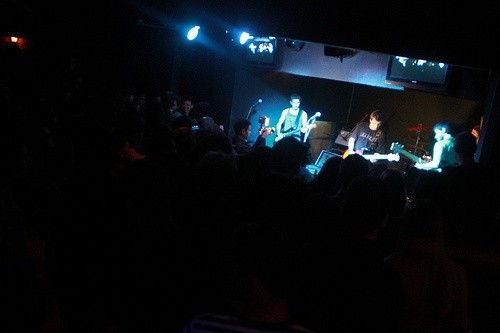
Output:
<box><xmin>444</xmin><ymin>131</ymin><xmax>478</xmax><ymax>187</ymax></box>
<box><xmin>275</xmin><ymin>93</ymin><xmax>308</xmax><ymax>142</ymax></box>
<box><xmin>231</xmin><ymin>119</ymin><xmax>252</xmax><ymax>152</ymax></box>
<box><xmin>182</xmin><ymin>96</ymin><xmax>193</xmax><ymax>115</ymax></box>
<box><xmin>418</xmin><ymin>123</ymin><xmax>455</xmax><ymax>169</ymax></box>
<box><xmin>342</xmin><ymin>109</ymin><xmax>387</xmax><ymax>160</ymax></box>
<box><xmin>0</xmin><ymin>89</ymin><xmax>499</xmax><ymax>333</ymax></box>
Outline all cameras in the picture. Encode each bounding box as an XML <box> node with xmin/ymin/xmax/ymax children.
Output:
<box><xmin>259</xmin><ymin>126</ymin><xmax>275</xmax><ymax>135</ymax></box>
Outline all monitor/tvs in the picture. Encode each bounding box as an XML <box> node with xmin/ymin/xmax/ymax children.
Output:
<box><xmin>244</xmin><ymin>35</ymin><xmax>276</xmax><ymax>68</ymax></box>
<box><xmin>386</xmin><ymin>55</ymin><xmax>452</xmax><ymax>89</ymax></box>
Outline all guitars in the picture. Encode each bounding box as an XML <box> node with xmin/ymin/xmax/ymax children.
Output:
<box><xmin>390</xmin><ymin>142</ymin><xmax>442</xmax><ymax>172</ymax></box>
<box><xmin>342</xmin><ymin>149</ymin><xmax>400</xmax><ymax>162</ymax></box>
<box><xmin>275</xmin><ymin>124</ymin><xmax>317</xmax><ymax>143</ymax></box>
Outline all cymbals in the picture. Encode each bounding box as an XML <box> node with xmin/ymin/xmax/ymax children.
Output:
<box><xmin>407</xmin><ymin>126</ymin><xmax>432</xmax><ymax>131</ymax></box>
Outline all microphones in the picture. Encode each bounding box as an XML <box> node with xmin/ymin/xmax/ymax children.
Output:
<box><xmin>252</xmin><ymin>99</ymin><xmax>262</xmax><ymax>107</ymax></box>
<box><xmin>308</xmin><ymin>112</ymin><xmax>321</xmax><ymax>121</ymax></box>
